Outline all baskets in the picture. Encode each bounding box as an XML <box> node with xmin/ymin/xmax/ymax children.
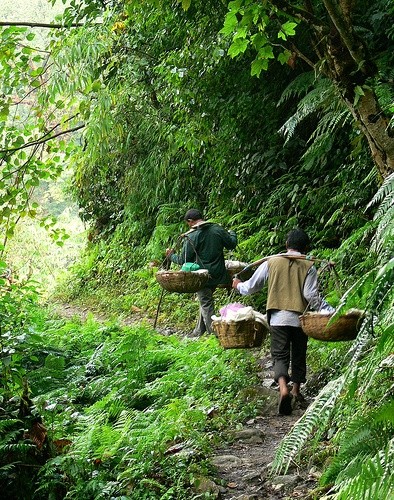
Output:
<box><xmin>211</xmin><ymin>316</ymin><xmax>271</xmax><ymax>350</ymax></box>
<box><xmin>154</xmin><ymin>267</ymin><xmax>209</xmax><ymax>293</ymax></box>
<box><xmin>223</xmin><ymin>266</ymin><xmax>253</xmax><ymax>282</ymax></box>
<box><xmin>298</xmin><ymin>310</ymin><xmax>362</xmax><ymax>342</ymax></box>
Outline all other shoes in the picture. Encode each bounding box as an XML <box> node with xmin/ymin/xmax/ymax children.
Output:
<box><xmin>278</xmin><ymin>393</ymin><xmax>305</xmax><ymax>416</ymax></box>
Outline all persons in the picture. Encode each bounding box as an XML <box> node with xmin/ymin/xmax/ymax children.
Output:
<box><xmin>166</xmin><ymin>209</ymin><xmax>237</xmax><ymax>336</ymax></box>
<box><xmin>233</xmin><ymin>229</ymin><xmax>334</xmax><ymax>415</ymax></box>
